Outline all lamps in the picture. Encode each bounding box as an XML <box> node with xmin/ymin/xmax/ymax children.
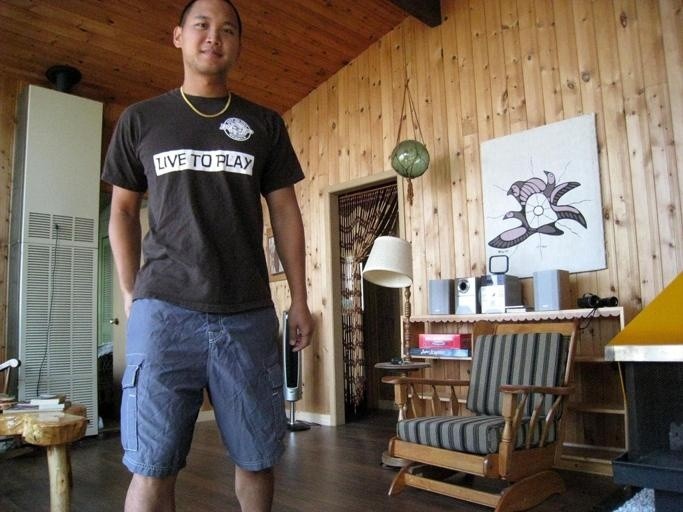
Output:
<box><xmin>359</xmin><ymin>236</ymin><xmax>414</xmax><ymax>363</ymax></box>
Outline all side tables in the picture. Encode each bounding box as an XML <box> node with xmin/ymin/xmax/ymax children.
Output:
<box><xmin>374</xmin><ymin>359</ymin><xmax>431</xmax><ymax>472</ymax></box>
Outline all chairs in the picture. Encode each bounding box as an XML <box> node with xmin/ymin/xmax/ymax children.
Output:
<box><xmin>380</xmin><ymin>317</ymin><xmax>578</xmax><ymax>512</ymax></box>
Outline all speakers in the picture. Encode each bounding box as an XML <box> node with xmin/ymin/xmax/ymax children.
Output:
<box><xmin>532</xmin><ymin>269</ymin><xmax>572</xmax><ymax>311</ymax></box>
<box><xmin>427</xmin><ymin>279</ymin><xmax>455</xmax><ymax>314</ymax></box>
<box><xmin>280</xmin><ymin>309</ymin><xmax>312</xmax><ymax>432</ymax></box>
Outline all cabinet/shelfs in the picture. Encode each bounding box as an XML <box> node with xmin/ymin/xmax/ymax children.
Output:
<box><xmin>399</xmin><ymin>305</ymin><xmax>632</xmax><ymax>476</ymax></box>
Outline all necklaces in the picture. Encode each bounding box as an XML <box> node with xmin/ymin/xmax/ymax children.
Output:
<box><xmin>180</xmin><ymin>86</ymin><xmax>232</xmax><ymax>118</ymax></box>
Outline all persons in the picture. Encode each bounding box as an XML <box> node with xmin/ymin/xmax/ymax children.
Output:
<box><xmin>100</xmin><ymin>1</ymin><xmax>316</xmax><ymax>512</ymax></box>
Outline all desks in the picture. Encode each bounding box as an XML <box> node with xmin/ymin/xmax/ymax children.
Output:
<box><xmin>0</xmin><ymin>396</ymin><xmax>87</xmax><ymax>512</ymax></box>
